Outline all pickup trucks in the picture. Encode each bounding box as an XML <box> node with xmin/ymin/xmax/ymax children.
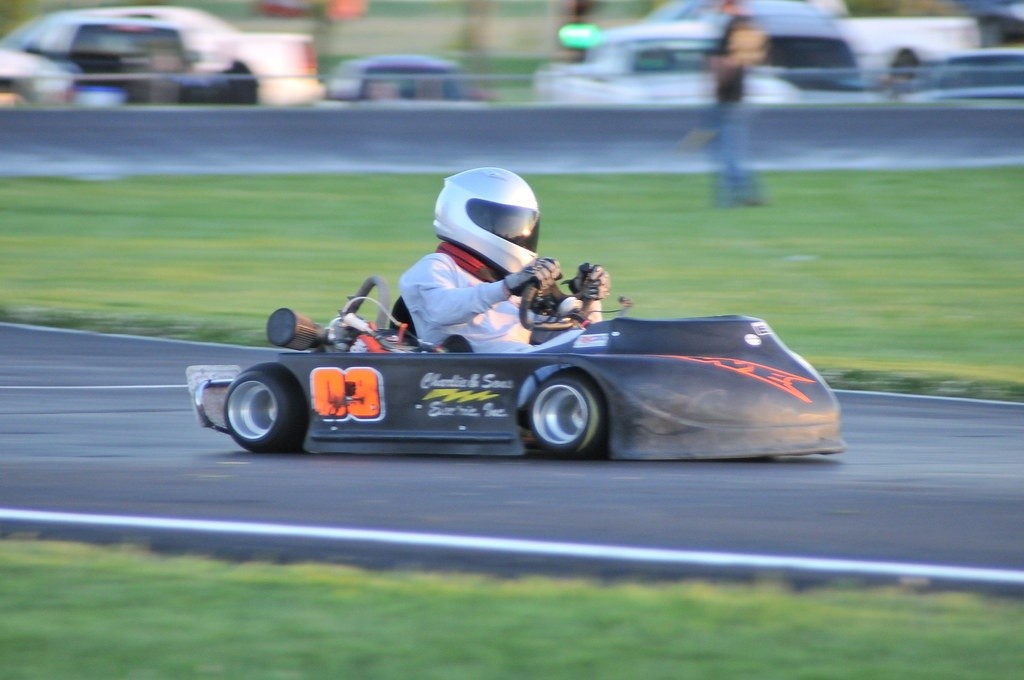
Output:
<box><xmin>329</xmin><ymin>55</ymin><xmax>493</xmax><ymax>100</ymax></box>
<box><xmin>535</xmin><ymin>0</ymin><xmax>980</xmax><ymax>103</ymax></box>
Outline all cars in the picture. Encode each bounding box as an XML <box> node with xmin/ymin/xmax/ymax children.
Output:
<box><xmin>0</xmin><ymin>6</ymin><xmax>318</xmax><ymax>104</ymax></box>
<box><xmin>901</xmin><ymin>47</ymin><xmax>1024</xmax><ymax>101</ymax></box>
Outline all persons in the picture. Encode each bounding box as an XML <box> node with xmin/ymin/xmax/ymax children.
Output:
<box><xmin>701</xmin><ymin>0</ymin><xmax>770</xmax><ymax>211</ymax></box>
<box><xmin>398</xmin><ymin>167</ymin><xmax>611</xmax><ymax>354</ymax></box>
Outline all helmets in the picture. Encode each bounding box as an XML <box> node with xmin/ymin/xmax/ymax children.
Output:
<box><xmin>433</xmin><ymin>167</ymin><xmax>540</xmax><ymax>276</ymax></box>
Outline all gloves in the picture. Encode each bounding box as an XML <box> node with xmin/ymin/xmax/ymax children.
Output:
<box><xmin>505</xmin><ymin>258</ymin><xmax>562</xmax><ymax>296</ymax></box>
<box><xmin>568</xmin><ymin>262</ymin><xmax>611</xmax><ymax>300</ymax></box>
